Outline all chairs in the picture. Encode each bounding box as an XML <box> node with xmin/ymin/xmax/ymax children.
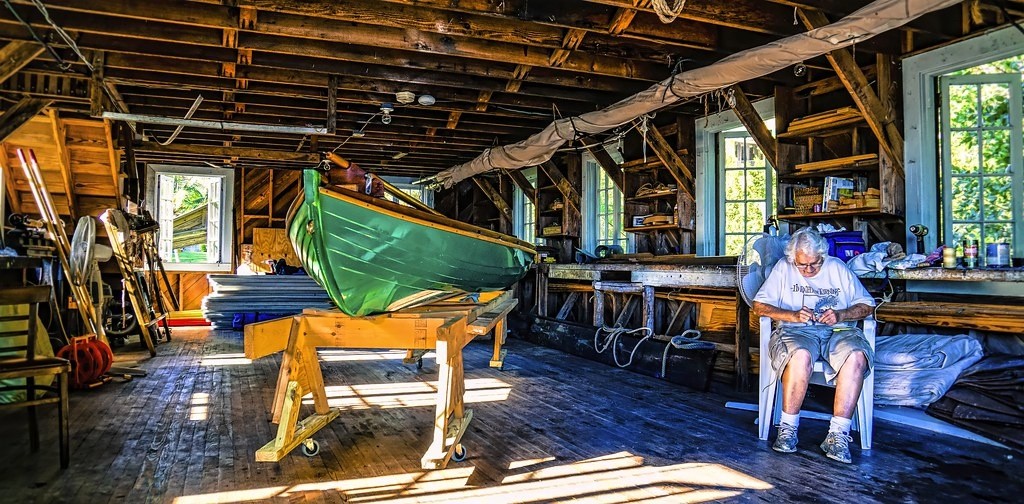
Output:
<box><xmin>759</xmin><ymin>314</ymin><xmax>877</xmax><ymax>448</ymax></box>
<box><xmin>0</xmin><ymin>284</ymin><xmax>71</xmax><ymax>468</ymax></box>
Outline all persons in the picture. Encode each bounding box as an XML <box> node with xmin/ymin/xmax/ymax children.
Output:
<box><xmin>753</xmin><ymin>229</ymin><xmax>877</xmax><ymax>464</ymax></box>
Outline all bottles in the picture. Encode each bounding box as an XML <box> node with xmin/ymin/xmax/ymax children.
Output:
<box><xmin>674</xmin><ymin>209</ymin><xmax>678</xmax><ymax>224</ymax></box>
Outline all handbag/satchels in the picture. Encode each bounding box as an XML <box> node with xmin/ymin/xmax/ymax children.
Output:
<box><xmin>820</xmin><ymin>231</ymin><xmax>892</xmax><ymax>277</ymax></box>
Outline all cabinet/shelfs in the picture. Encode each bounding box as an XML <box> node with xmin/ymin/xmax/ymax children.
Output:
<box><xmin>776</xmin><ymin>52</ymin><xmax>902</xmax><ymax>223</ymax></box>
<box><xmin>535</xmin><ymin>152</ymin><xmax>582</xmax><ymax>238</ymax></box>
<box><xmin>622</xmin><ymin>115</ymin><xmax>697</xmax><ymax>231</ymax></box>
<box><xmin>472</xmin><ymin>174</ymin><xmax>512</xmax><ymax>233</ymax></box>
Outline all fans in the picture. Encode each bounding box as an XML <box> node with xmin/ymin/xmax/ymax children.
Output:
<box><xmin>71</xmin><ymin>216</ymin><xmax>147</xmax><ymax>380</ymax></box>
<box><xmin>725</xmin><ymin>233</ymin><xmax>833</xmax><ymax>425</ymax></box>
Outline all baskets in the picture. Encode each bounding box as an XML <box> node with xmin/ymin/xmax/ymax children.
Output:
<box><xmin>794</xmin><ymin>187</ymin><xmax>823</xmax><ymax>215</ymax></box>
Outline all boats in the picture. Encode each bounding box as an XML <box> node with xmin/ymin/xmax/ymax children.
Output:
<box><xmin>283</xmin><ymin>170</ymin><xmax>540</xmax><ymax>318</ymax></box>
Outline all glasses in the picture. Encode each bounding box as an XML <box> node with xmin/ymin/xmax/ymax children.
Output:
<box><xmin>794</xmin><ymin>258</ymin><xmax>824</xmax><ymax>269</ymax></box>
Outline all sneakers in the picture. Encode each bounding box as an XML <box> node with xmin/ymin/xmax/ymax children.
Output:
<box><xmin>820</xmin><ymin>427</ymin><xmax>853</xmax><ymax>464</ymax></box>
<box><xmin>772</xmin><ymin>422</ymin><xmax>799</xmax><ymax>453</ymax></box>
